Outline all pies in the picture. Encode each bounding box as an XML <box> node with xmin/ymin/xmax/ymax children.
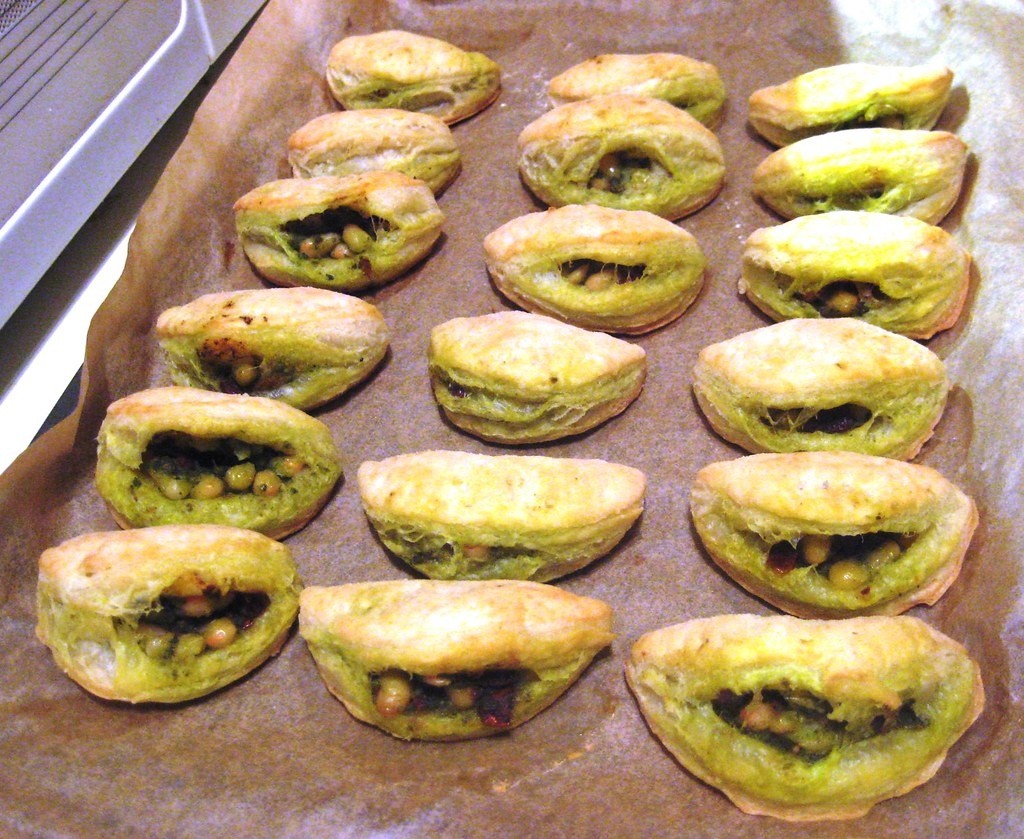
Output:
<box><xmin>32</xmin><ymin>30</ymin><xmax>985</xmax><ymax>824</ymax></box>
